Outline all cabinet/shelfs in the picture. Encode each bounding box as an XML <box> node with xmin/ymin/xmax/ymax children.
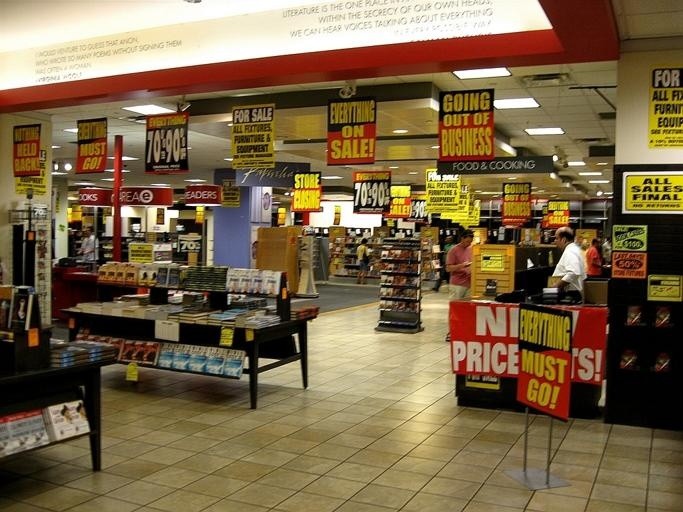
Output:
<box><xmin>420</xmin><ymin>226</ymin><xmax>544</xmax><ymax>282</ymax></box>
<box><xmin>68</xmin><ymin>217</ymin><xmax>207</xmax><ymax>266</ymax></box>
<box><xmin>56</xmin><ymin>272</ymin><xmax>319</xmax><ymax>410</ymax></box>
<box><xmin>374</xmin><ymin>238</ymin><xmax>424</xmax><ymax>335</ymax></box>
<box><xmin>255</xmin><ymin>225</ymin><xmax>389</xmax><ymax>298</ymax></box>
<box><xmin>0</xmin><ymin>323</ymin><xmax>117</xmax><ymax>471</ymax></box>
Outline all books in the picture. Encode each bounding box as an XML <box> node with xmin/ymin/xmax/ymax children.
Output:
<box><xmin>378</xmin><ymin>248</ymin><xmax>421</xmax><ymax>313</ymax></box>
<box><xmin>0</xmin><ymin>261</ymin><xmax>281</xmax><ymax>380</ymax></box>
<box><xmin>0</xmin><ymin>397</ymin><xmax>91</xmax><ymax>459</ymax></box>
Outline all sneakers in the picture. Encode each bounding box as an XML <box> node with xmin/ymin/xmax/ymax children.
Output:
<box><xmin>445</xmin><ymin>332</ymin><xmax>450</xmax><ymax>342</ymax></box>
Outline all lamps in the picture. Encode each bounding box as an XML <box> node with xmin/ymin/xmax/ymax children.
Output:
<box><xmin>338</xmin><ymin>80</ymin><xmax>356</xmax><ymax>100</ymax></box>
<box><xmin>177</xmin><ymin>95</ymin><xmax>192</xmax><ymax>112</ymax></box>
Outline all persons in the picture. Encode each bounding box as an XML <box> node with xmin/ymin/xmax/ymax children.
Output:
<box><xmin>548</xmin><ymin>225</ymin><xmax>588</xmax><ymax>306</ymax></box>
<box><xmin>430</xmin><ymin>234</ymin><xmax>456</xmax><ymax>294</ymax></box>
<box><xmin>75</xmin><ymin>226</ymin><xmax>99</xmax><ymax>261</ymax></box>
<box><xmin>67</xmin><ymin>228</ymin><xmax>76</xmax><ymax>259</ymax></box>
<box><xmin>445</xmin><ymin>228</ymin><xmax>474</xmax><ymax>342</ymax></box>
<box><xmin>574</xmin><ymin>234</ymin><xmax>611</xmax><ymax>279</ymax></box>
<box><xmin>356</xmin><ymin>238</ymin><xmax>372</xmax><ymax>284</ymax></box>
